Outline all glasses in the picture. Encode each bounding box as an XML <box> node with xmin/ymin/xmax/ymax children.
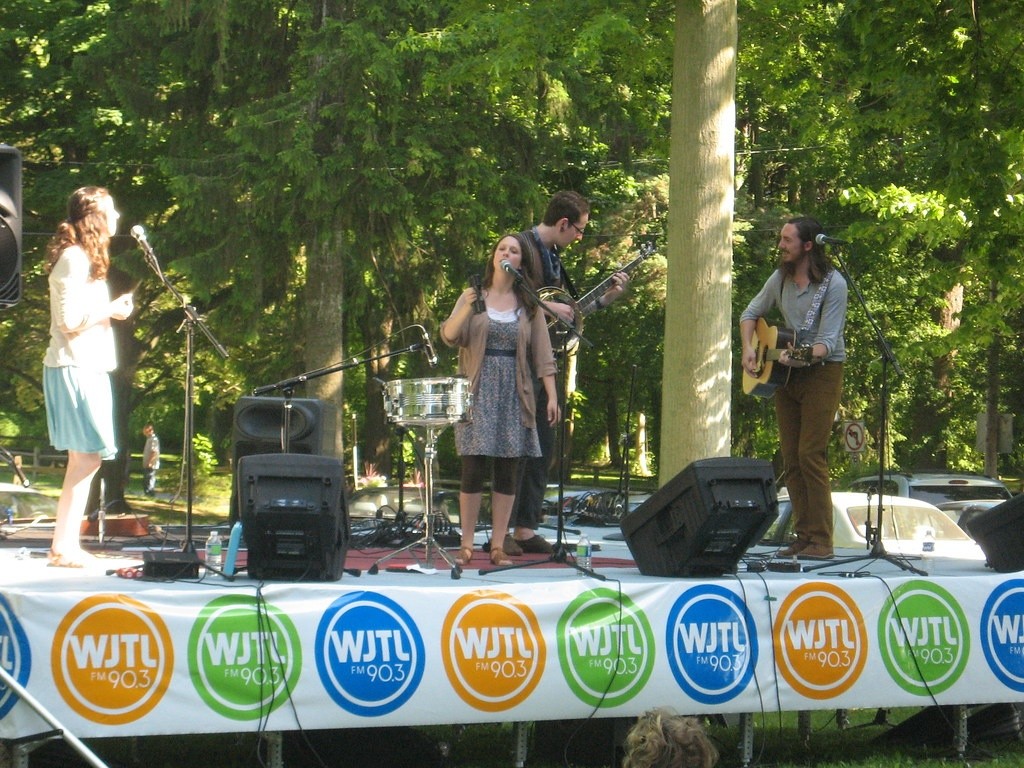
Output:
<box><xmin>570</xmin><ymin>221</ymin><xmax>586</xmax><ymax>237</ymax></box>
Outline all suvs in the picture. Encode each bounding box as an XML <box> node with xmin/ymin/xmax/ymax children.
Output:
<box><xmin>849</xmin><ymin>470</ymin><xmax>1014</xmax><ymax>525</ymax></box>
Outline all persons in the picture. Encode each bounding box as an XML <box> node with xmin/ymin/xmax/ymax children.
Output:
<box><xmin>623</xmin><ymin>707</ymin><xmax>719</xmax><ymax>768</ymax></box>
<box><xmin>39</xmin><ymin>184</ymin><xmax>135</xmax><ymax>569</ymax></box>
<box><xmin>739</xmin><ymin>216</ymin><xmax>848</xmax><ymax>561</ymax></box>
<box><xmin>482</xmin><ymin>190</ymin><xmax>631</xmax><ymax>557</ymax></box>
<box><xmin>141</xmin><ymin>424</ymin><xmax>161</xmax><ymax>498</ymax></box>
<box><xmin>439</xmin><ymin>234</ymin><xmax>561</xmax><ymax>566</ymax></box>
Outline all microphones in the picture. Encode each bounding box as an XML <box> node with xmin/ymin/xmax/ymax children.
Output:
<box><xmin>500</xmin><ymin>260</ymin><xmax>526</xmax><ymax>281</ymax></box>
<box><xmin>816</xmin><ymin>234</ymin><xmax>852</xmax><ymax>244</ymax></box>
<box><xmin>131</xmin><ymin>226</ymin><xmax>158</xmax><ymax>264</ymax></box>
<box><xmin>421</xmin><ymin>326</ymin><xmax>439</xmax><ymax>368</ymax></box>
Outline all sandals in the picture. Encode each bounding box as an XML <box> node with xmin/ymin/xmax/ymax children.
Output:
<box><xmin>490</xmin><ymin>547</ymin><xmax>513</xmax><ymax>566</ymax></box>
<box><xmin>46</xmin><ymin>545</ymin><xmax>109</xmax><ymax>568</ymax></box>
<box><xmin>454</xmin><ymin>548</ymin><xmax>472</xmax><ymax>566</ymax></box>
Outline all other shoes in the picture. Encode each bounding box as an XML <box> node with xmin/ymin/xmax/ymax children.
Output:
<box><xmin>796</xmin><ymin>541</ymin><xmax>834</xmax><ymax>559</ymax></box>
<box><xmin>778</xmin><ymin>539</ymin><xmax>806</xmax><ymax>558</ymax></box>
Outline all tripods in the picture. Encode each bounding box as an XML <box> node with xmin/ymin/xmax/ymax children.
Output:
<box><xmin>802</xmin><ymin>243</ymin><xmax>928</xmax><ymax>576</ymax></box>
<box><xmin>482</xmin><ymin>279</ymin><xmax>606</xmax><ymax>581</ymax></box>
<box><xmin>369</xmin><ymin>427</ymin><xmax>462</xmax><ymax>580</ymax></box>
<box><xmin>106</xmin><ymin>254</ymin><xmax>236</xmax><ymax>583</ymax></box>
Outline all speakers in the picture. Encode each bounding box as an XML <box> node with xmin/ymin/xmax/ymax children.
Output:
<box><xmin>619</xmin><ymin>456</ymin><xmax>781</xmax><ymax>578</ymax></box>
<box><xmin>967</xmin><ymin>492</ymin><xmax>1024</xmax><ymax>573</ymax></box>
<box><xmin>0</xmin><ymin>145</ymin><xmax>21</xmax><ymax>310</ymax></box>
<box><xmin>227</xmin><ymin>396</ymin><xmax>353</xmax><ymax>581</ymax></box>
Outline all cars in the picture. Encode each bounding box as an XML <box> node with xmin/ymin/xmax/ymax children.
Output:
<box><xmin>757</xmin><ymin>492</ymin><xmax>992</xmax><ymax>568</ymax></box>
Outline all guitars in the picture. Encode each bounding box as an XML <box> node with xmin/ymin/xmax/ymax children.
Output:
<box><xmin>742</xmin><ymin>318</ymin><xmax>814</xmax><ymax>399</ymax></box>
<box><xmin>536</xmin><ymin>241</ymin><xmax>659</xmax><ymax>355</ymax></box>
<box><xmin>1</xmin><ymin>513</ymin><xmax>150</xmax><ymax>537</ymax></box>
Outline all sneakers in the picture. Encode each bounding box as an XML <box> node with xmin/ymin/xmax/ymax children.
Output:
<box><xmin>516</xmin><ymin>532</ymin><xmax>554</xmax><ymax>553</ymax></box>
<box><xmin>490</xmin><ymin>534</ymin><xmax>523</xmax><ymax>556</ymax></box>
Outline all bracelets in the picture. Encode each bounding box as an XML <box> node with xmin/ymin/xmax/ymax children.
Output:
<box><xmin>595</xmin><ymin>296</ymin><xmax>604</xmax><ymax>310</ymax></box>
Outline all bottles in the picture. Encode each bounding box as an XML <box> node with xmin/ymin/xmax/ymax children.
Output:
<box><xmin>921</xmin><ymin>530</ymin><xmax>935</xmax><ymax>570</ymax></box>
<box><xmin>205</xmin><ymin>531</ymin><xmax>226</xmax><ymax>573</ymax></box>
<box><xmin>6</xmin><ymin>505</ymin><xmax>12</xmax><ymax>525</ymax></box>
<box><xmin>576</xmin><ymin>534</ymin><xmax>592</xmax><ymax>577</ymax></box>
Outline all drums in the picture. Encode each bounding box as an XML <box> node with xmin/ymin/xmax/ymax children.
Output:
<box><xmin>381</xmin><ymin>376</ymin><xmax>474</xmax><ymax>427</ymax></box>
